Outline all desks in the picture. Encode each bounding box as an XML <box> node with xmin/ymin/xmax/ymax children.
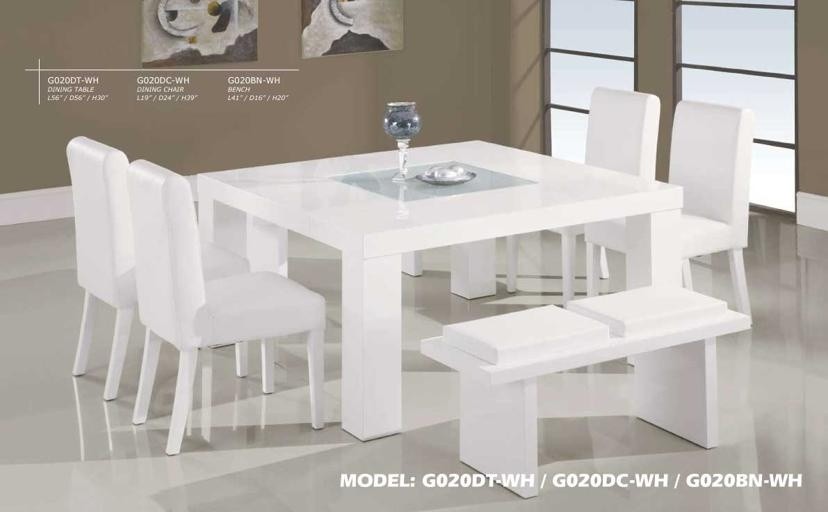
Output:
<box><xmin>196</xmin><ymin>136</ymin><xmax>685</xmax><ymax>443</ymax></box>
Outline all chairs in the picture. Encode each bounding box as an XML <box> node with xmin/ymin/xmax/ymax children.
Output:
<box><xmin>507</xmin><ymin>85</ymin><xmax>754</xmax><ymax>326</ymax></box>
<box><xmin>67</xmin><ymin>135</ymin><xmax>327</xmax><ymax>456</ymax></box>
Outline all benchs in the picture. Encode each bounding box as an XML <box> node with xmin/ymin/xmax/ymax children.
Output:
<box><xmin>420</xmin><ymin>284</ymin><xmax>752</xmax><ymax>499</ymax></box>
<box><xmin>244</xmin><ymin>215</ymin><xmax>421</xmax><ymax>277</ymax></box>
